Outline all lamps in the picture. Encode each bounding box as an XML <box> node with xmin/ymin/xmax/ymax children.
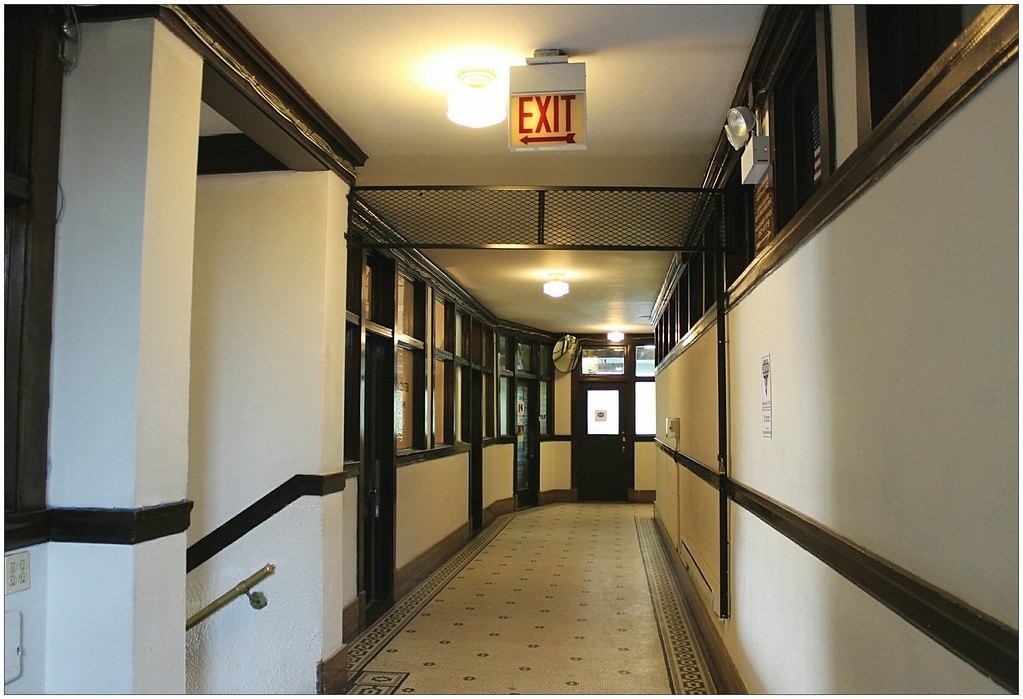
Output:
<box><xmin>446</xmin><ymin>69</ymin><xmax>507</xmax><ymax>130</ymax></box>
<box><xmin>543</xmin><ymin>273</ymin><xmax>570</xmax><ymax>298</ymax></box>
<box><xmin>606</xmin><ymin>328</ymin><xmax>625</xmax><ymax>343</ymax></box>
<box><xmin>722</xmin><ymin>107</ymin><xmax>772</xmax><ymax>186</ymax></box>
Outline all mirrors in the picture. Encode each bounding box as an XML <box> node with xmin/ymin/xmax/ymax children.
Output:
<box><xmin>551</xmin><ymin>334</ymin><xmax>582</xmax><ymax>373</ymax></box>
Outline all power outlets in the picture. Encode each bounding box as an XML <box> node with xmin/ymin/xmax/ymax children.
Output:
<box><xmin>4</xmin><ymin>550</ymin><xmax>31</xmax><ymax>596</ymax></box>
